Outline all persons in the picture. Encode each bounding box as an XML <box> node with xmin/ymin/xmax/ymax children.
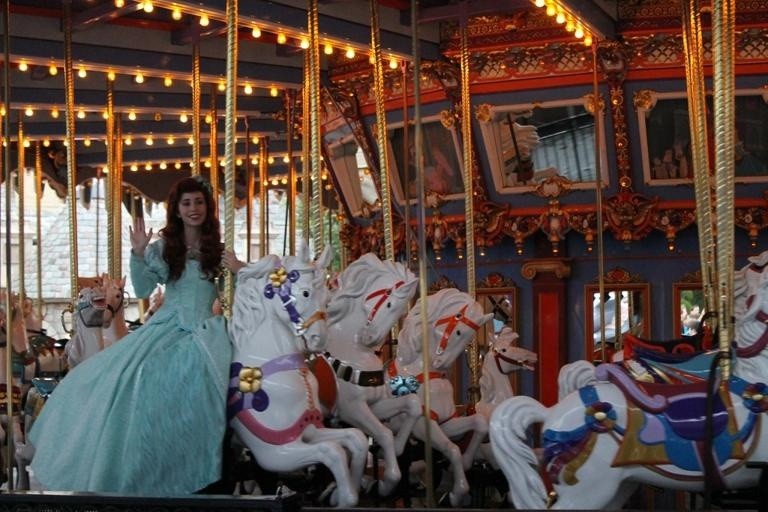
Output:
<box><xmin>27</xmin><ymin>178</ymin><xmax>249</xmax><ymax>497</ymax></box>
<box><xmin>680</xmin><ymin>302</ymin><xmax>706</xmax><ymax>337</ymax></box>
